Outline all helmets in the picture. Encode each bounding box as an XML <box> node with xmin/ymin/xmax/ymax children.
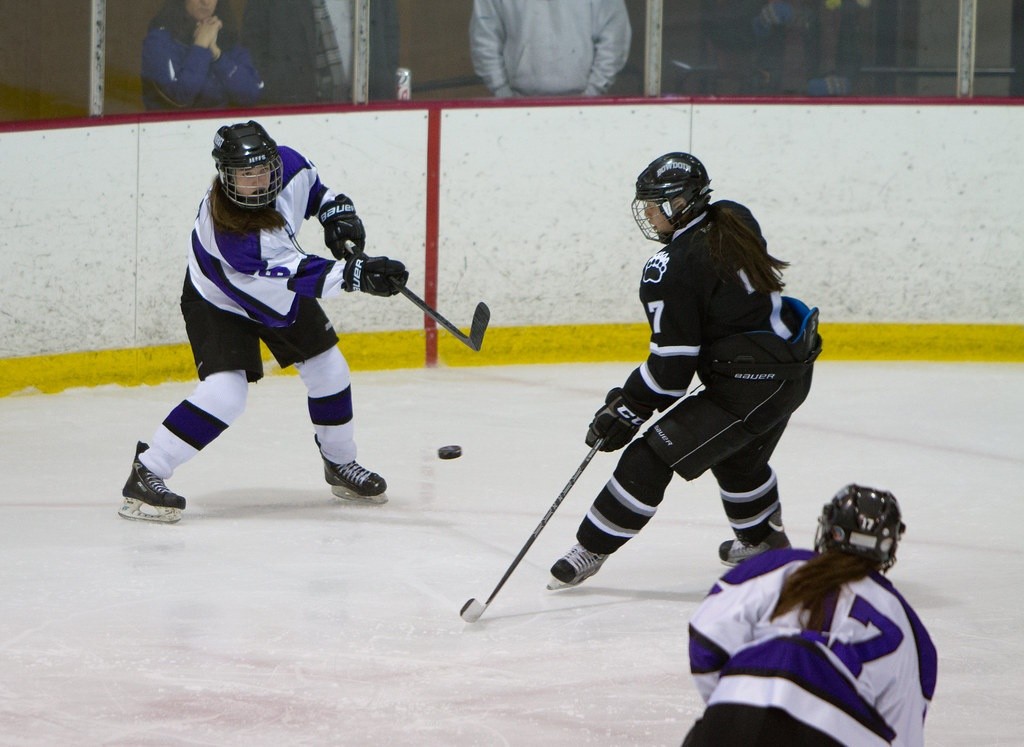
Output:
<box><xmin>815</xmin><ymin>484</ymin><xmax>906</xmax><ymax>573</ymax></box>
<box><xmin>631</xmin><ymin>152</ymin><xmax>714</xmax><ymax>244</ymax></box>
<box><xmin>212</xmin><ymin>120</ymin><xmax>283</xmax><ymax>210</ymax></box>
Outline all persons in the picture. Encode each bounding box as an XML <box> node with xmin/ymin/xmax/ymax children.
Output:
<box><xmin>543</xmin><ymin>151</ymin><xmax>823</xmax><ymax>594</ymax></box>
<box><xmin>680</xmin><ymin>484</ymin><xmax>936</xmax><ymax>747</ymax></box>
<box><xmin>141</xmin><ymin>0</ymin><xmax>274</xmax><ymax>109</ymax></box>
<box><xmin>700</xmin><ymin>0</ymin><xmax>863</xmax><ymax>94</ymax></box>
<box><xmin>470</xmin><ymin>0</ymin><xmax>630</xmax><ymax>99</ymax></box>
<box><xmin>119</xmin><ymin>122</ymin><xmax>410</xmax><ymax>522</ymax></box>
<box><xmin>243</xmin><ymin>0</ymin><xmax>402</xmax><ymax>106</ymax></box>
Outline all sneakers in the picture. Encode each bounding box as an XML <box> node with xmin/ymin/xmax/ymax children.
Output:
<box><xmin>719</xmin><ymin>502</ymin><xmax>792</xmax><ymax>578</ymax></box>
<box><xmin>547</xmin><ymin>543</ymin><xmax>609</xmax><ymax>590</ymax></box>
<box><xmin>314</xmin><ymin>433</ymin><xmax>388</xmax><ymax>505</ymax></box>
<box><xmin>118</xmin><ymin>441</ymin><xmax>186</xmax><ymax>524</ymax></box>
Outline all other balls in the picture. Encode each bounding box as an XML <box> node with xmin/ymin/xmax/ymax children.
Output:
<box><xmin>437</xmin><ymin>445</ymin><xmax>463</xmax><ymax>458</ymax></box>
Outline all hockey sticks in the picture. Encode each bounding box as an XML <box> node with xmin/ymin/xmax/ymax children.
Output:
<box><xmin>341</xmin><ymin>236</ymin><xmax>491</xmax><ymax>350</ymax></box>
<box><xmin>459</xmin><ymin>436</ymin><xmax>604</xmax><ymax>624</ymax></box>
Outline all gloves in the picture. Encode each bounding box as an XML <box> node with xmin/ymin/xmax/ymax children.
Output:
<box><xmin>586</xmin><ymin>388</ymin><xmax>653</xmax><ymax>452</ymax></box>
<box><xmin>319</xmin><ymin>194</ymin><xmax>366</xmax><ymax>261</ymax></box>
<box><xmin>345</xmin><ymin>257</ymin><xmax>408</xmax><ymax>297</ymax></box>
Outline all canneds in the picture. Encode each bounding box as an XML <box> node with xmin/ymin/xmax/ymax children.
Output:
<box><xmin>395</xmin><ymin>67</ymin><xmax>412</xmax><ymax>101</ymax></box>
